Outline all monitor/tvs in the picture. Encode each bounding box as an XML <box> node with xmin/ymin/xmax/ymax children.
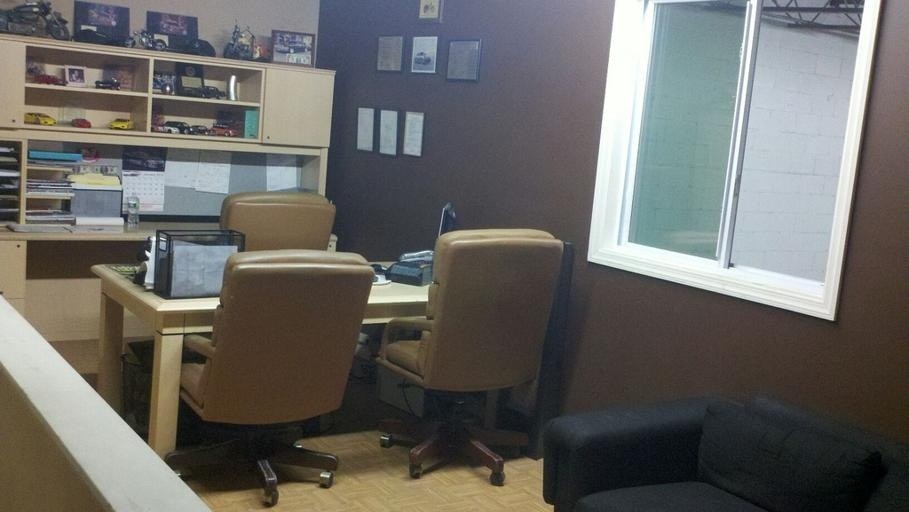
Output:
<box><xmin>438</xmin><ymin>201</ymin><xmax>472</xmax><ymax>238</ymax></box>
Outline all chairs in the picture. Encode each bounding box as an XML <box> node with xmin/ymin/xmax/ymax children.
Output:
<box><xmin>181</xmin><ymin>248</ymin><xmax>377</xmax><ymax>505</ymax></box>
<box><xmin>544</xmin><ymin>398</ymin><xmax>909</xmax><ymax>512</ymax></box>
<box><xmin>378</xmin><ymin>228</ymin><xmax>565</xmax><ymax>485</ymax></box>
<box><xmin>221</xmin><ymin>192</ymin><xmax>338</xmax><ymax>252</ymax></box>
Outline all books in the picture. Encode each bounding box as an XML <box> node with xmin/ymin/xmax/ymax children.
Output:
<box><xmin>0</xmin><ymin>147</ymin><xmax>124</xmax><ymax>234</ymax></box>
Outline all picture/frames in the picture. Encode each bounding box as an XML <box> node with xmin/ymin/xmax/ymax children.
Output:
<box><xmin>64</xmin><ymin>64</ymin><xmax>88</xmax><ymax>87</ymax></box>
<box><xmin>446</xmin><ymin>39</ymin><xmax>482</xmax><ymax>82</ymax></box>
<box><xmin>416</xmin><ymin>0</ymin><xmax>444</xmax><ymax>23</ymax></box>
<box><xmin>411</xmin><ymin>36</ymin><xmax>438</xmax><ymax>74</ymax></box>
<box><xmin>268</xmin><ymin>29</ymin><xmax>314</xmax><ymax>67</ymax></box>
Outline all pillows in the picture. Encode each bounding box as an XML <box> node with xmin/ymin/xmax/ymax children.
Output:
<box><xmin>698</xmin><ymin>397</ymin><xmax>886</xmax><ymax>512</ymax></box>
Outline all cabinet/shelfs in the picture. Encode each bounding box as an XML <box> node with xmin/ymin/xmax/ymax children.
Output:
<box><xmin>0</xmin><ymin>225</ymin><xmax>338</xmax><ymax>342</ymax></box>
<box><xmin>0</xmin><ymin>34</ymin><xmax>337</xmax><ymax>225</ymax></box>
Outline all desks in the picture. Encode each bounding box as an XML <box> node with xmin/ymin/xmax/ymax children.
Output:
<box><xmin>89</xmin><ymin>262</ymin><xmax>428</xmax><ymax>460</ymax></box>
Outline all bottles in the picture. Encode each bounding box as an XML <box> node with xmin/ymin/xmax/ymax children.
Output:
<box><xmin>127</xmin><ymin>192</ymin><xmax>140</xmax><ymax>232</ymax></box>
